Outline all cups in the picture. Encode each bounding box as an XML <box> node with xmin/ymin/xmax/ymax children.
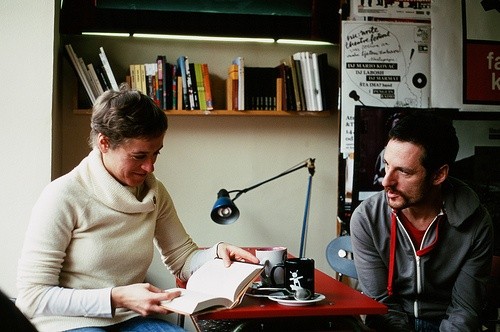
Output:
<box><xmin>270</xmin><ymin>258</ymin><xmax>314</xmax><ymax>300</ymax></box>
<box><xmin>256</xmin><ymin>247</ymin><xmax>287</xmax><ymax>286</ymax></box>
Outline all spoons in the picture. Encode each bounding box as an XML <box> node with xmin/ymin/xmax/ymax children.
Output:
<box><xmin>257</xmin><ymin>288</ymin><xmax>311</xmax><ymax>300</ymax></box>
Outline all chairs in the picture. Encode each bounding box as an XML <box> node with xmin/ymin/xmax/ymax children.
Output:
<box><xmin>326</xmin><ymin>235</ymin><xmax>359</xmax><ymax>289</ymax></box>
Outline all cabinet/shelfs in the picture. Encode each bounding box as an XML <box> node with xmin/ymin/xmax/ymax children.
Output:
<box><xmin>61</xmin><ymin>0</ymin><xmax>346</xmax><ymax>119</ymax></box>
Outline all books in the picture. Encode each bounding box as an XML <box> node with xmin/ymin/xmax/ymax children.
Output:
<box><xmin>65</xmin><ymin>43</ymin><xmax>331</xmax><ymax>111</ymax></box>
<box><xmin>156</xmin><ymin>257</ymin><xmax>266</xmax><ymax>316</ymax></box>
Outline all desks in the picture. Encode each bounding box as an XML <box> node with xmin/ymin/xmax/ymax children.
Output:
<box><xmin>176</xmin><ymin>247</ymin><xmax>388</xmax><ymax>332</ymax></box>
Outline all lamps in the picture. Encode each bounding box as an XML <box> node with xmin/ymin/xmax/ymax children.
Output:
<box><xmin>210</xmin><ymin>157</ymin><xmax>316</xmax><ymax>259</ymax></box>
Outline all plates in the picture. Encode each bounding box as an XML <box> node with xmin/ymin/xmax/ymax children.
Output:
<box><xmin>245</xmin><ymin>281</ymin><xmax>284</xmax><ymax>297</ymax></box>
<box><xmin>268</xmin><ymin>292</ymin><xmax>325</xmax><ymax>306</ymax></box>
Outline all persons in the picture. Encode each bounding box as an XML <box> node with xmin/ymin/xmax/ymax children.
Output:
<box><xmin>350</xmin><ymin>112</ymin><xmax>494</xmax><ymax>332</ymax></box>
<box><xmin>15</xmin><ymin>90</ymin><xmax>260</xmax><ymax>332</ymax></box>
